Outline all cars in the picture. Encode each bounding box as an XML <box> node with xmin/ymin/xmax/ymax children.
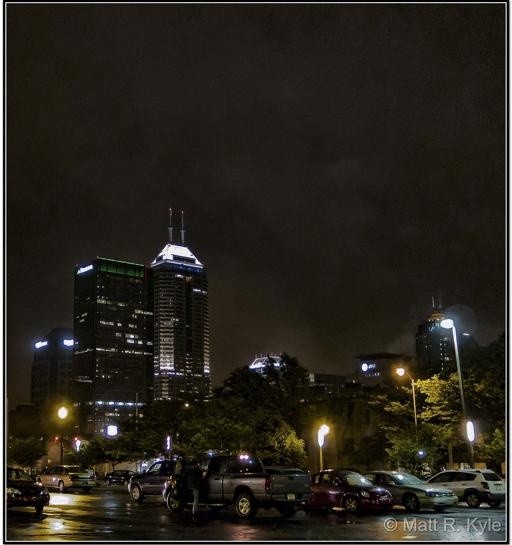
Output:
<box><xmin>36</xmin><ymin>465</ymin><xmax>96</xmax><ymax>492</ymax></box>
<box><xmin>7</xmin><ymin>468</ymin><xmax>50</xmax><ymax>512</ymax></box>
<box><xmin>128</xmin><ymin>456</ymin><xmax>504</xmax><ymax>519</ymax></box>
<box><xmin>109</xmin><ymin>470</ymin><xmax>134</xmax><ymax>485</ymax></box>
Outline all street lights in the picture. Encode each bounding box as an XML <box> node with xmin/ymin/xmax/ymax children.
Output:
<box><xmin>396</xmin><ymin>369</ymin><xmax>418</xmax><ymax>440</ymax></box>
<box><xmin>58</xmin><ymin>407</ymin><xmax>68</xmax><ymax>464</ymax></box>
<box><xmin>318</xmin><ymin>425</ymin><xmax>328</xmax><ymax>470</ymax></box>
<box><xmin>441</xmin><ymin>319</ymin><xmax>474</xmax><ymax>462</ymax></box>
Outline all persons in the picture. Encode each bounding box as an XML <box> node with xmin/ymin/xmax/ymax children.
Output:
<box><xmin>187</xmin><ymin>462</ymin><xmax>201</xmax><ymax>517</ymax></box>
